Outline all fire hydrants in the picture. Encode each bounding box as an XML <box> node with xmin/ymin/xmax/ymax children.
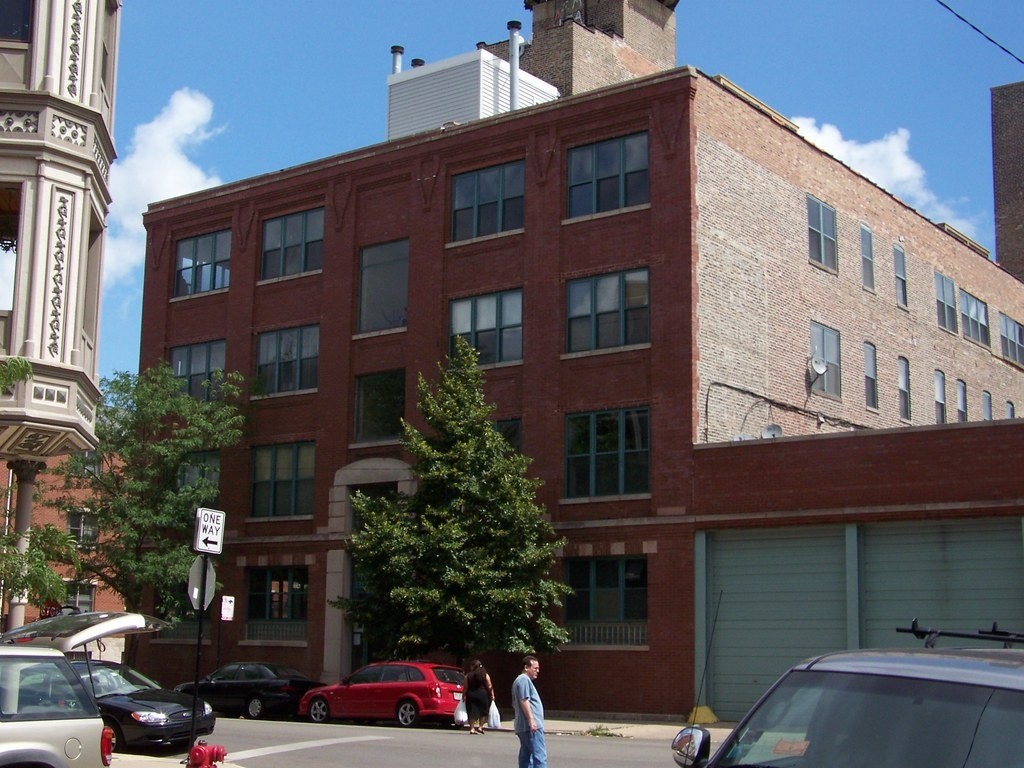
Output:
<box><xmin>180</xmin><ymin>740</ymin><xmax>227</xmax><ymax>768</ymax></box>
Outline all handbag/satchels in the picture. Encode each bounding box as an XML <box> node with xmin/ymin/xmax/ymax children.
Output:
<box><xmin>454</xmin><ymin>698</ymin><xmax>468</xmax><ymax>725</ymax></box>
<box><xmin>488</xmin><ymin>700</ymin><xmax>501</xmax><ymax>728</ymax></box>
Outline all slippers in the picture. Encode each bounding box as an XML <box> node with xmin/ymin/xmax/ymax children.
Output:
<box><xmin>470</xmin><ymin>732</ymin><xmax>477</xmax><ymax>734</ymax></box>
<box><xmin>477</xmin><ymin>729</ymin><xmax>484</xmax><ymax>734</ymax></box>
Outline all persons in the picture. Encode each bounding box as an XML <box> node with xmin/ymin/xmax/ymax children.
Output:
<box><xmin>462</xmin><ymin>660</ymin><xmax>495</xmax><ymax>734</ymax></box>
<box><xmin>512</xmin><ymin>656</ymin><xmax>548</xmax><ymax>768</ymax></box>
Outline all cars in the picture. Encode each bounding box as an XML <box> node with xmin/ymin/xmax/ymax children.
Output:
<box><xmin>174</xmin><ymin>662</ymin><xmax>326</xmax><ymax>720</ymax></box>
<box><xmin>299</xmin><ymin>661</ymin><xmax>467</xmax><ymax>727</ymax></box>
<box><xmin>17</xmin><ymin>659</ymin><xmax>216</xmax><ymax>752</ymax></box>
<box><xmin>0</xmin><ymin>611</ymin><xmax>174</xmax><ymax>768</ymax></box>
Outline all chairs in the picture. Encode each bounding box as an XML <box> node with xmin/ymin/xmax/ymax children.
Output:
<box><xmin>383</xmin><ymin>672</ymin><xmax>399</xmax><ymax>681</ymax></box>
<box><xmin>85</xmin><ymin>676</ymin><xmax>103</xmax><ymax>695</ymax></box>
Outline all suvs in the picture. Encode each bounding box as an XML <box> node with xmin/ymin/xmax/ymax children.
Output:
<box><xmin>671</xmin><ymin>619</ymin><xmax>1024</xmax><ymax>768</ymax></box>
